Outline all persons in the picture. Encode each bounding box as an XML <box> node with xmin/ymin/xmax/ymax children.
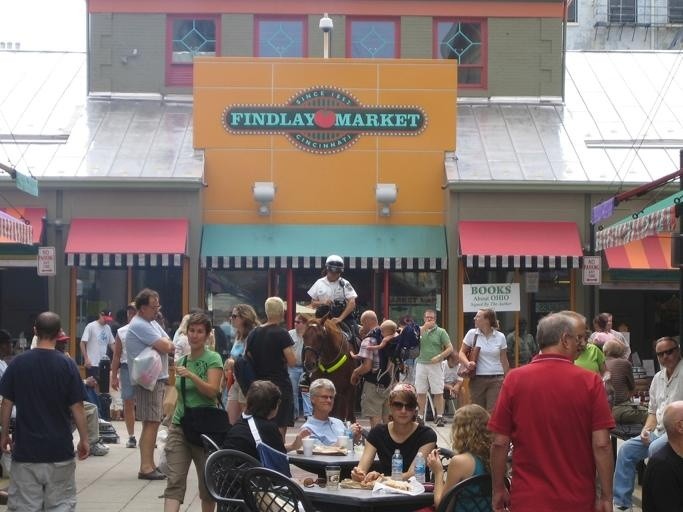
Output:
<box><xmin>1</xmin><ymin>255</ymin><xmax>635</xmax><ymax>456</ymax></box>
<box><xmin>125</xmin><ymin>288</ymin><xmax>174</xmax><ymax>480</ymax></box>
<box><xmin>425</xmin><ymin>404</ymin><xmax>490</xmax><ymax>511</ymax></box>
<box><xmin>614</xmin><ymin>337</ymin><xmax>683</xmax><ymax>512</ymax></box>
<box><xmin>640</xmin><ymin>400</ymin><xmax>682</xmax><ymax>511</ymax></box>
<box><xmin>163</xmin><ymin>312</ymin><xmax>223</xmax><ymax>510</ymax></box>
<box><xmin>221</xmin><ymin>381</ymin><xmax>309</xmax><ymax>466</ymax></box>
<box><xmin>0</xmin><ymin>311</ymin><xmax>90</xmax><ymax>511</ymax></box>
<box><xmin>349</xmin><ymin>382</ymin><xmax>436</xmax><ymax>482</ymax></box>
<box><xmin>492</xmin><ymin>314</ymin><xmax>614</xmax><ymax>510</ymax></box>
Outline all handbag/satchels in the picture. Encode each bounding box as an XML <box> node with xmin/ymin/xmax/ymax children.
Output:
<box><xmin>457</xmin><ymin>346</ymin><xmax>481</xmax><ymax>377</ymax></box>
<box><xmin>233</xmin><ymin>354</ymin><xmax>260</xmax><ymax>395</ymax></box>
<box><xmin>255</xmin><ymin>442</ymin><xmax>292</xmax><ymax>477</ymax></box>
<box><xmin>181</xmin><ymin>406</ymin><xmax>230</xmax><ymax>447</ymax></box>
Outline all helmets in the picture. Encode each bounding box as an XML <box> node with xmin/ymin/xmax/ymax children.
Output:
<box><xmin>325</xmin><ymin>255</ymin><xmax>344</xmax><ymax>272</ymax></box>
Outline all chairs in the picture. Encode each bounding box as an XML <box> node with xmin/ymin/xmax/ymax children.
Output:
<box><xmin>610</xmin><ymin>404</ymin><xmax>648</xmax><ymax>485</ymax></box>
<box><xmin>242</xmin><ymin>467</ymin><xmax>316</xmax><ymax>512</ymax></box>
<box><xmin>438</xmin><ymin>471</ymin><xmax>510</xmax><ymax>512</ymax></box>
<box><xmin>198</xmin><ymin>432</ymin><xmax>222</xmax><ymax>456</ymax></box>
<box><xmin>203</xmin><ymin>448</ymin><xmax>263</xmax><ymax>511</ymax></box>
<box><xmin>425</xmin><ymin>445</ymin><xmax>457</xmax><ymax>483</ymax></box>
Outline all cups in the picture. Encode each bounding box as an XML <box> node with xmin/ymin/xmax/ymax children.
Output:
<box><xmin>335</xmin><ymin>436</ymin><xmax>349</xmax><ymax>448</ymax></box>
<box><xmin>324</xmin><ymin>465</ymin><xmax>340</xmax><ymax>490</ymax></box>
<box><xmin>300</xmin><ymin>438</ymin><xmax>315</xmax><ymax>456</ymax></box>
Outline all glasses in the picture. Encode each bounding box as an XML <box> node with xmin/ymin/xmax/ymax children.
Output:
<box><xmin>304</xmin><ymin>478</ymin><xmax>326</xmax><ymax>488</ymax></box>
<box><xmin>392</xmin><ymin>401</ymin><xmax>416</xmax><ymax>411</ymax></box>
<box><xmin>656</xmin><ymin>348</ymin><xmax>674</xmax><ymax>356</ymax></box>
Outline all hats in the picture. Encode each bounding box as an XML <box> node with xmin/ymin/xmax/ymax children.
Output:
<box><xmin>100</xmin><ymin>308</ymin><xmax>112</xmax><ymax>322</ymax></box>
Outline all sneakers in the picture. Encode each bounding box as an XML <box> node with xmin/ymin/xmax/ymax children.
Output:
<box><xmin>90</xmin><ymin>436</ymin><xmax>166</xmax><ymax>479</ymax></box>
<box><xmin>434</xmin><ymin>416</ymin><xmax>444</xmax><ymax>427</ymax></box>
<box><xmin>417</xmin><ymin>415</ymin><xmax>424</xmax><ymax>426</ymax></box>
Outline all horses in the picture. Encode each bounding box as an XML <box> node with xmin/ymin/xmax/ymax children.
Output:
<box><xmin>298</xmin><ymin>311</ymin><xmax>360</xmax><ymax>423</ymax></box>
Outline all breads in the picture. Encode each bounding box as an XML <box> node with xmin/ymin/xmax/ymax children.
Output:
<box><xmin>387</xmin><ymin>483</ymin><xmax>413</xmax><ymax>492</ymax></box>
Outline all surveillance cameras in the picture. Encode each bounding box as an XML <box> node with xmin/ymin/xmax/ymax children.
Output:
<box><xmin>319</xmin><ymin>19</ymin><xmax>334</xmax><ymax>33</ymax></box>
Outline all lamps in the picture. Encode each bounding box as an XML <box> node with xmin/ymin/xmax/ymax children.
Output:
<box><xmin>252</xmin><ymin>179</ymin><xmax>278</xmax><ymax>217</ymax></box>
<box><xmin>374</xmin><ymin>184</ymin><xmax>398</xmax><ymax>217</ymax></box>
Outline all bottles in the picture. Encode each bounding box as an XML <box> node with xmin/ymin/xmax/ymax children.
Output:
<box><xmin>413</xmin><ymin>451</ymin><xmax>425</xmax><ymax>482</ymax></box>
<box><xmin>391</xmin><ymin>449</ymin><xmax>403</xmax><ymax>480</ymax></box>
<box><xmin>343</xmin><ymin>421</ymin><xmax>353</xmax><ymax>452</ymax></box>
